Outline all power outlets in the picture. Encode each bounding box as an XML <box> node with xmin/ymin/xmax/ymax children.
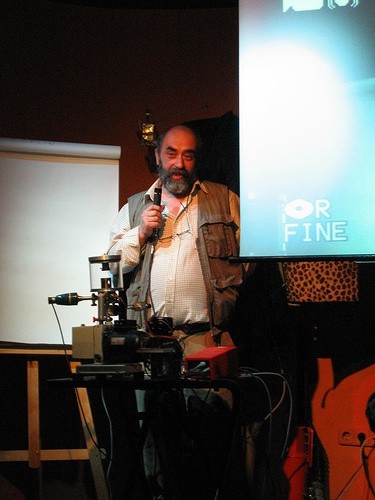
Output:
<box><xmin>339</xmin><ymin>430</ymin><xmax>374</xmax><ymax>447</ymax></box>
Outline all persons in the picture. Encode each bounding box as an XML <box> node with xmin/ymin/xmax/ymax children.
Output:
<box><xmin>106</xmin><ymin>125</ymin><xmax>259</xmax><ymax>413</ymax></box>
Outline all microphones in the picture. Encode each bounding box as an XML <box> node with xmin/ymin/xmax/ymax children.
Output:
<box><xmin>152</xmin><ymin>188</ymin><xmax>162</xmax><ymax>246</ymax></box>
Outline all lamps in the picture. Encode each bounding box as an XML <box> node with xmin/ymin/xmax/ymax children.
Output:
<box><xmin>278</xmin><ymin>261</ymin><xmax>359</xmax><ymax>370</ymax></box>
<box><xmin>135</xmin><ymin>108</ymin><xmax>157</xmax><ymax>147</ymax></box>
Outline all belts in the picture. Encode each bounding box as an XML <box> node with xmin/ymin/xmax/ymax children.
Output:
<box><xmin>172</xmin><ymin>322</ymin><xmax>210</xmax><ymax>333</ymax></box>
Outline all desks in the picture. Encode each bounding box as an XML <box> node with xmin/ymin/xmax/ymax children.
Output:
<box><xmin>47</xmin><ymin>374</ymin><xmax>286</xmax><ymax>499</ymax></box>
